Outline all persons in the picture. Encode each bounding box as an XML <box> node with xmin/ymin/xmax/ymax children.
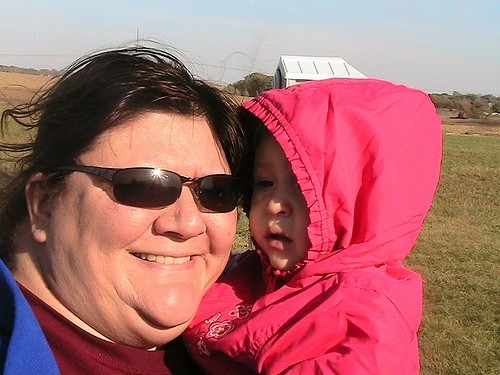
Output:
<box><xmin>178</xmin><ymin>78</ymin><xmax>442</xmax><ymax>375</ymax></box>
<box><xmin>0</xmin><ymin>46</ymin><xmax>247</xmax><ymax>375</ymax></box>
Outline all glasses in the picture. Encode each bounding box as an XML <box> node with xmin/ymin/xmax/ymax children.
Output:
<box><xmin>48</xmin><ymin>161</ymin><xmax>247</xmax><ymax>215</ymax></box>
